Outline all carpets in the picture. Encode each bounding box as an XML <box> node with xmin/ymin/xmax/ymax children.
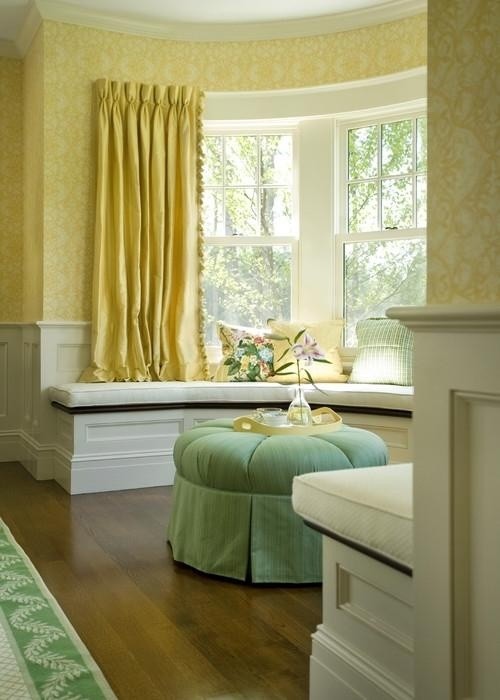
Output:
<box><xmin>0</xmin><ymin>517</ymin><xmax>118</xmax><ymax>700</ymax></box>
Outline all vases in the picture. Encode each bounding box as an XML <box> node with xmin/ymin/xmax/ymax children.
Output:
<box><xmin>286</xmin><ymin>387</ymin><xmax>311</xmax><ymax>426</ymax></box>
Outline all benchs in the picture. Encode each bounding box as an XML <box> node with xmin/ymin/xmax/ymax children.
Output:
<box><xmin>48</xmin><ymin>382</ymin><xmax>413</xmax><ymax>699</ymax></box>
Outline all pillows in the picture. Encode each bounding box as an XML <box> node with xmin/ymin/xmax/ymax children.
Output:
<box><xmin>348</xmin><ymin>316</ymin><xmax>413</xmax><ymax>385</ymax></box>
<box><xmin>212</xmin><ymin>319</ymin><xmax>275</xmax><ymax>381</ymax></box>
<box><xmin>267</xmin><ymin>316</ymin><xmax>349</xmax><ymax>382</ymax></box>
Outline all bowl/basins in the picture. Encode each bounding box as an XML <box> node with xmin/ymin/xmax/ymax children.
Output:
<box><xmin>255</xmin><ymin>407</ymin><xmax>289</xmax><ymax>426</ymax></box>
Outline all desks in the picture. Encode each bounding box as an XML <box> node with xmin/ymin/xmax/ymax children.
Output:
<box><xmin>167</xmin><ymin>414</ymin><xmax>390</xmax><ymax>586</ymax></box>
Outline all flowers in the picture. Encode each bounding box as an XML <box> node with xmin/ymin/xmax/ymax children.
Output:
<box><xmin>217</xmin><ymin>323</ymin><xmax>274</xmax><ymax>382</ymax></box>
<box><xmin>262</xmin><ymin>325</ymin><xmax>333</xmax><ymax>419</ymax></box>
